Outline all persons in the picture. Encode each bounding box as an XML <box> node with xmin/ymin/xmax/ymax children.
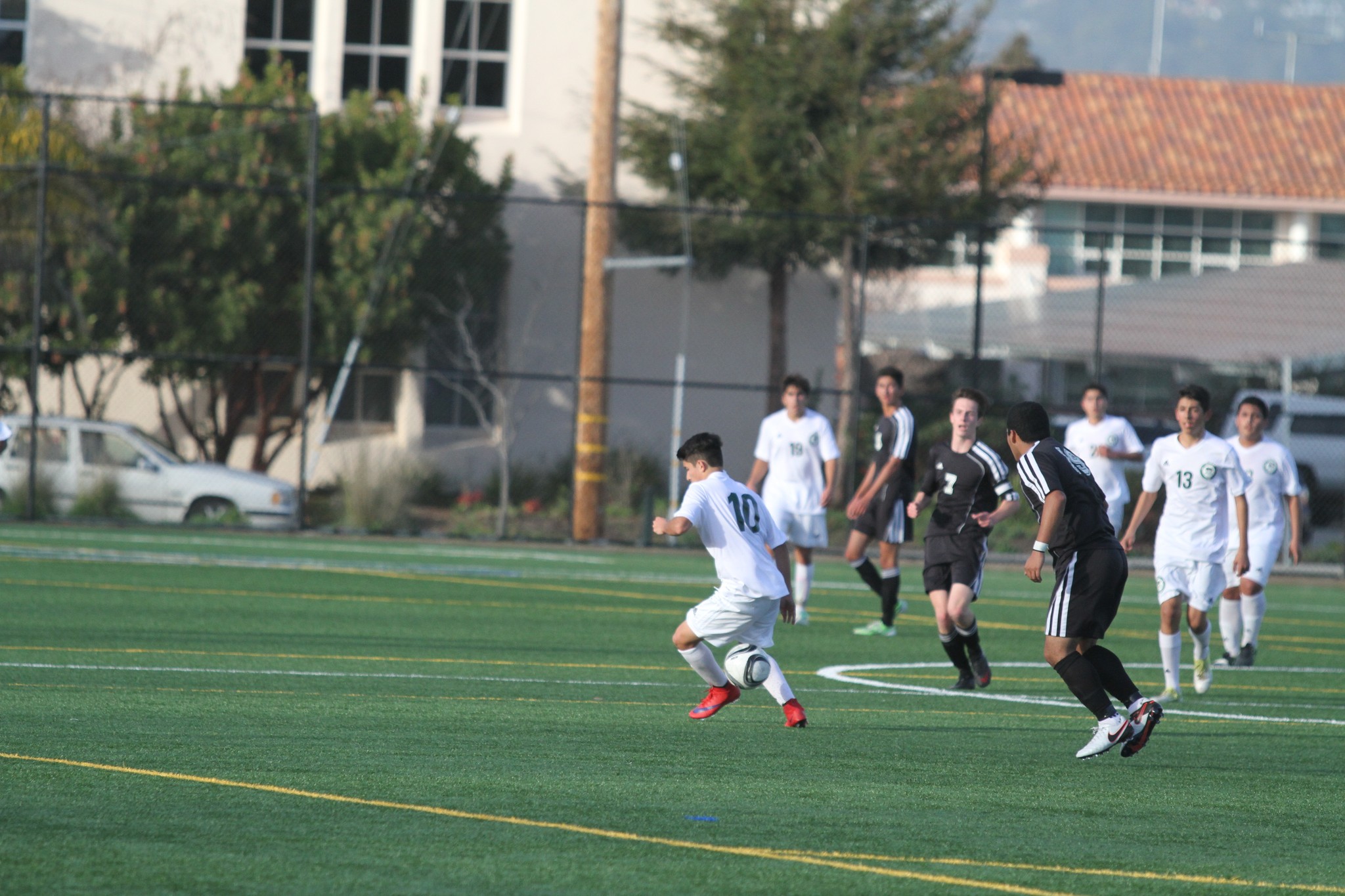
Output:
<box><xmin>745</xmin><ymin>374</ymin><xmax>841</xmax><ymax>622</ymax></box>
<box><xmin>844</xmin><ymin>365</ymin><xmax>914</xmax><ymax>637</ymax></box>
<box><xmin>1214</xmin><ymin>396</ymin><xmax>1303</xmax><ymax>668</ymax></box>
<box><xmin>1118</xmin><ymin>383</ymin><xmax>1253</xmax><ymax>703</ymax></box>
<box><xmin>1064</xmin><ymin>384</ymin><xmax>1146</xmax><ymax>539</ymax></box>
<box><xmin>906</xmin><ymin>388</ymin><xmax>1022</xmax><ymax>690</ymax></box>
<box><xmin>653</xmin><ymin>433</ymin><xmax>807</xmax><ymax>729</ymax></box>
<box><xmin>1006</xmin><ymin>401</ymin><xmax>1164</xmax><ymax>760</ymax></box>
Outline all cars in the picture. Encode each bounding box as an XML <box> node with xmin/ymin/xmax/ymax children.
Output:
<box><xmin>0</xmin><ymin>414</ymin><xmax>301</xmax><ymax>531</ymax></box>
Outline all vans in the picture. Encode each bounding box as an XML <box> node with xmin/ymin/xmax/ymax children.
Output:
<box><xmin>1052</xmin><ymin>416</ymin><xmax>1180</xmax><ymax>469</ymax></box>
<box><xmin>1224</xmin><ymin>389</ymin><xmax>1344</xmax><ymax>495</ymax></box>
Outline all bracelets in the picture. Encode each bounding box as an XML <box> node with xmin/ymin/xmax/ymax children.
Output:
<box><xmin>1032</xmin><ymin>540</ymin><xmax>1049</xmax><ymax>553</ymax></box>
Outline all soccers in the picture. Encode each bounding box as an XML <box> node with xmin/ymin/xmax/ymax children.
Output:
<box><xmin>724</xmin><ymin>644</ymin><xmax>771</xmax><ymax>691</ymax></box>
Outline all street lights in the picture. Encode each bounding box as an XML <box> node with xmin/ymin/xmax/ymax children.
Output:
<box><xmin>971</xmin><ymin>65</ymin><xmax>1066</xmax><ymax>388</ymax></box>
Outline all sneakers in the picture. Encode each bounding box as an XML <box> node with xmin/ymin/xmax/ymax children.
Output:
<box><xmin>1234</xmin><ymin>643</ymin><xmax>1257</xmax><ymax>667</ymax></box>
<box><xmin>1215</xmin><ymin>652</ymin><xmax>1235</xmax><ymax>666</ymax></box>
<box><xmin>1076</xmin><ymin>715</ymin><xmax>1134</xmax><ymax>760</ymax></box>
<box><xmin>951</xmin><ymin>676</ymin><xmax>975</xmax><ymax>690</ymax></box>
<box><xmin>1193</xmin><ymin>650</ymin><xmax>1212</xmax><ymax>695</ymax></box>
<box><xmin>795</xmin><ymin>608</ymin><xmax>808</xmax><ymax>628</ymax></box>
<box><xmin>1149</xmin><ymin>688</ymin><xmax>1182</xmax><ymax>703</ymax></box>
<box><xmin>783</xmin><ymin>699</ymin><xmax>808</xmax><ymax>728</ymax></box>
<box><xmin>853</xmin><ymin>620</ymin><xmax>897</xmax><ymax>637</ymax></box>
<box><xmin>895</xmin><ymin>601</ymin><xmax>909</xmax><ymax>619</ymax></box>
<box><xmin>689</xmin><ymin>680</ymin><xmax>741</xmax><ymax>719</ymax></box>
<box><xmin>970</xmin><ymin>653</ymin><xmax>992</xmax><ymax>688</ymax></box>
<box><xmin>1120</xmin><ymin>698</ymin><xmax>1164</xmax><ymax>758</ymax></box>
